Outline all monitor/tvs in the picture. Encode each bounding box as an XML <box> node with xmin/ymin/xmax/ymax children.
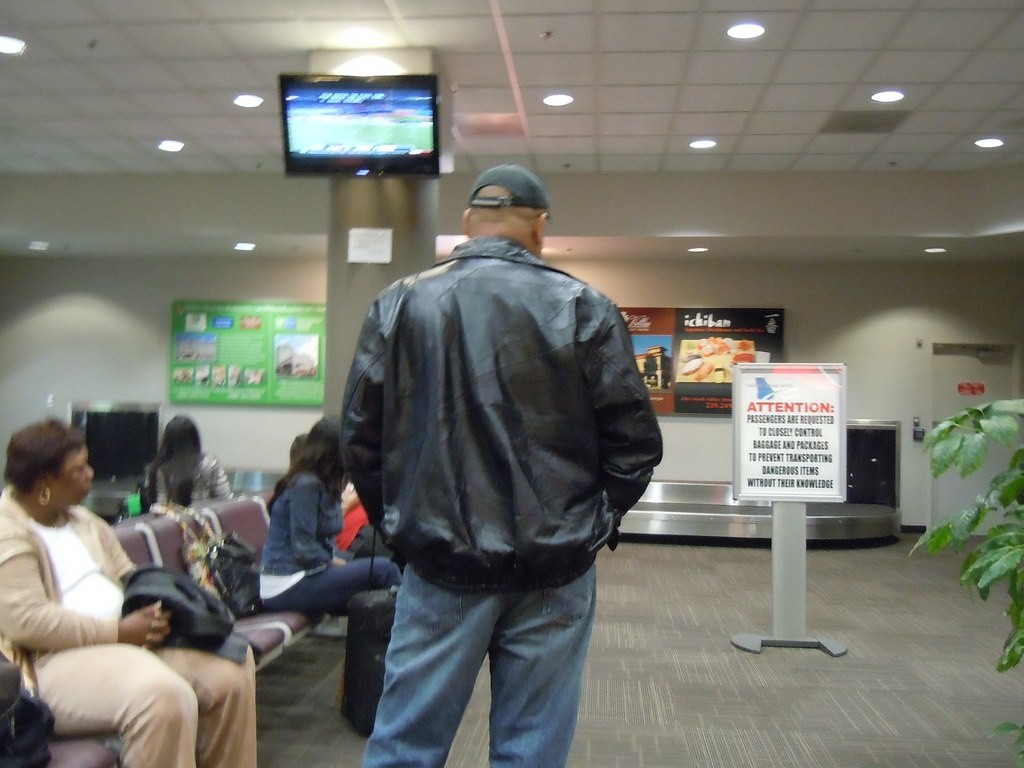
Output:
<box><xmin>278</xmin><ymin>73</ymin><xmax>439</xmax><ymax>177</ymax></box>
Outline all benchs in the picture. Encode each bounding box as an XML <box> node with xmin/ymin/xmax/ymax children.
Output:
<box><xmin>0</xmin><ymin>491</ymin><xmax>315</xmax><ymax>768</ymax></box>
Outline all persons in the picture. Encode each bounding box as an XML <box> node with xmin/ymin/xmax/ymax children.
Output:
<box><xmin>0</xmin><ymin>417</ymin><xmax>258</xmax><ymax>768</ymax></box>
<box><xmin>341</xmin><ymin>164</ymin><xmax>663</xmax><ymax>768</ymax></box>
<box><xmin>145</xmin><ymin>416</ymin><xmax>231</xmax><ymax>508</ymax></box>
<box><xmin>259</xmin><ymin>414</ymin><xmax>402</xmax><ymax>738</ymax></box>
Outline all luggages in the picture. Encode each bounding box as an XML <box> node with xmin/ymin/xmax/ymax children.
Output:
<box><xmin>340</xmin><ymin>586</ymin><xmax>400</xmax><ymax>736</ymax></box>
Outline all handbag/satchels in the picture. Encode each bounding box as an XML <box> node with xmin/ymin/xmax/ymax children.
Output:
<box><xmin>207</xmin><ymin>531</ymin><xmax>262</xmax><ymax>618</ymax></box>
<box><xmin>142</xmin><ymin>466</ymin><xmax>158</xmax><ymax>513</ymax></box>
<box><xmin>151</xmin><ymin>502</ymin><xmax>236</xmax><ymax>624</ymax></box>
<box><xmin>0</xmin><ymin>690</ymin><xmax>55</xmax><ymax>768</ymax></box>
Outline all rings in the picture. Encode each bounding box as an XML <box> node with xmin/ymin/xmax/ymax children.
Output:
<box><xmin>150</xmin><ymin>620</ymin><xmax>159</xmax><ymax>630</ymax></box>
<box><xmin>145</xmin><ymin>632</ymin><xmax>152</xmax><ymax>643</ymax></box>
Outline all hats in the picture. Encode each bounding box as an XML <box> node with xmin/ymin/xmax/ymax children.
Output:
<box><xmin>467</xmin><ymin>164</ymin><xmax>549</xmax><ymax>208</ymax></box>
